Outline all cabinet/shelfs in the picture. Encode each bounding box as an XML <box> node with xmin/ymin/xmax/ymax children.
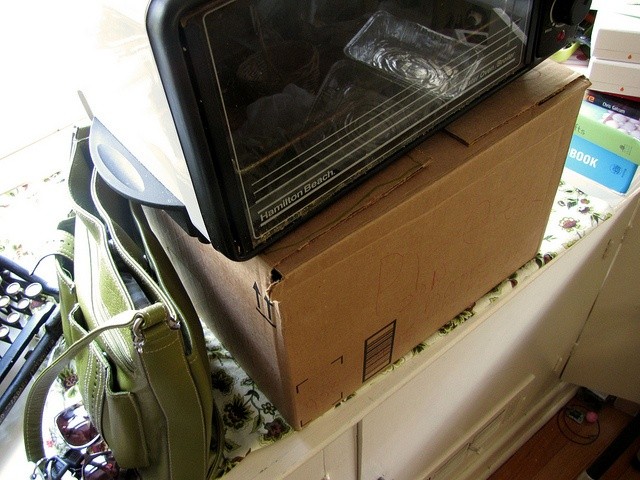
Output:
<box><xmin>221</xmin><ymin>196</ymin><xmax>640</xmax><ymax>480</ymax></box>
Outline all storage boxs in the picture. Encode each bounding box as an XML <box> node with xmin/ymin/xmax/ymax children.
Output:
<box><xmin>139</xmin><ymin>59</ymin><xmax>593</xmax><ymax>433</ymax></box>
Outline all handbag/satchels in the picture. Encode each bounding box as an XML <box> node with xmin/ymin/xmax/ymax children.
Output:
<box><xmin>24</xmin><ymin>125</ymin><xmax>225</xmax><ymax>480</ymax></box>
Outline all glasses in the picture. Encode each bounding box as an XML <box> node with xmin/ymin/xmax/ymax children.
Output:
<box><xmin>54</xmin><ymin>400</ymin><xmax>137</xmax><ymax>479</ymax></box>
